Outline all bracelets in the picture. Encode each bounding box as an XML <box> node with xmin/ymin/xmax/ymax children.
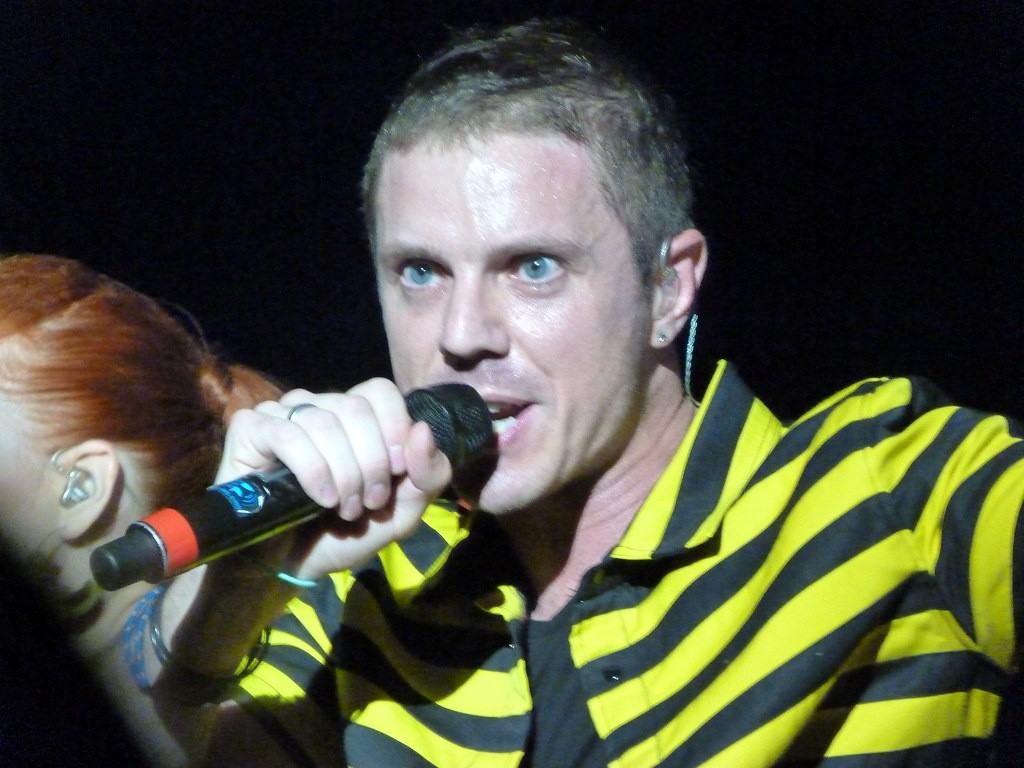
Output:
<box><xmin>236</xmin><ymin>550</ymin><xmax>320</xmax><ymax>589</ymax></box>
<box><xmin>122</xmin><ymin>580</ymin><xmax>271</xmax><ymax>708</ymax></box>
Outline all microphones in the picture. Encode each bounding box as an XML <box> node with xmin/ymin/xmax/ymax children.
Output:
<box><xmin>87</xmin><ymin>383</ymin><xmax>493</xmax><ymax>591</ymax></box>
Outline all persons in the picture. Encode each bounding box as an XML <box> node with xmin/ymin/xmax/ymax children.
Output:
<box><xmin>0</xmin><ymin>253</ymin><xmax>286</xmax><ymax>768</ymax></box>
<box><xmin>141</xmin><ymin>18</ymin><xmax>1024</xmax><ymax>768</ymax></box>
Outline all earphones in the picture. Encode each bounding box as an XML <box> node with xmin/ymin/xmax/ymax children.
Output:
<box><xmin>657</xmin><ymin>266</ymin><xmax>681</xmax><ymax>315</ymax></box>
<box><xmin>59</xmin><ymin>467</ymin><xmax>97</xmax><ymax>508</ymax></box>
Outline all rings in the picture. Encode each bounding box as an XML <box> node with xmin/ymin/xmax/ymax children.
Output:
<box><xmin>286</xmin><ymin>402</ymin><xmax>316</xmax><ymax>423</ymax></box>
